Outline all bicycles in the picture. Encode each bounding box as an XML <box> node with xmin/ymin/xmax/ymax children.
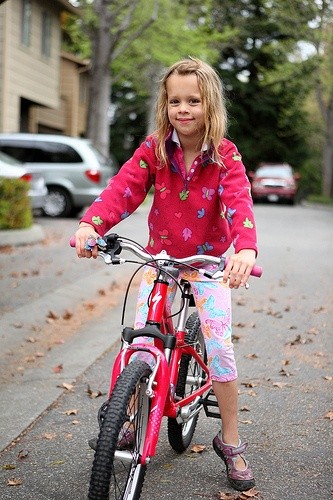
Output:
<box><xmin>67</xmin><ymin>233</ymin><xmax>266</xmax><ymax>500</ymax></box>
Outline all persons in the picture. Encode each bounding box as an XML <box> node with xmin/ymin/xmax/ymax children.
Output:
<box><xmin>73</xmin><ymin>56</ymin><xmax>259</xmax><ymax>493</ymax></box>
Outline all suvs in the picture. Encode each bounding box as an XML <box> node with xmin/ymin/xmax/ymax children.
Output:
<box><xmin>248</xmin><ymin>161</ymin><xmax>302</xmax><ymax>205</ymax></box>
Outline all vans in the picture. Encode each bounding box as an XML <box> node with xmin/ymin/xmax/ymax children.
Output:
<box><xmin>0</xmin><ymin>134</ymin><xmax>115</xmax><ymax>216</ymax></box>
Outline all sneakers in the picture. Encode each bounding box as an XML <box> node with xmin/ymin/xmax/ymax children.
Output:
<box><xmin>89</xmin><ymin>424</ymin><xmax>136</xmax><ymax>451</ymax></box>
<box><xmin>213</xmin><ymin>433</ymin><xmax>255</xmax><ymax>490</ymax></box>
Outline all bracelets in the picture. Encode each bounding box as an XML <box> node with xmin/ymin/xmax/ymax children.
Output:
<box><xmin>77</xmin><ymin>224</ymin><xmax>95</xmax><ymax>230</ymax></box>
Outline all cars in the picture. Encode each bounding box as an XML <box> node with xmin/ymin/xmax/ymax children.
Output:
<box><xmin>0</xmin><ymin>154</ymin><xmax>48</xmax><ymax>210</ymax></box>
